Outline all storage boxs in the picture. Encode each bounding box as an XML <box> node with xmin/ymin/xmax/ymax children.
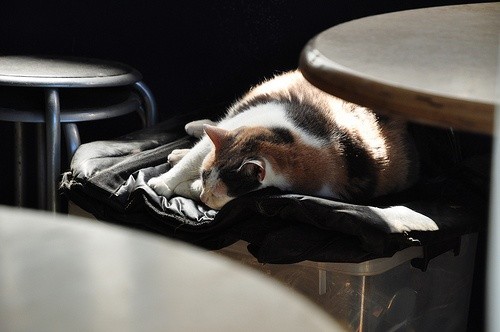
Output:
<box><xmin>208</xmin><ymin>220</ymin><xmax>480</xmax><ymax>330</ymax></box>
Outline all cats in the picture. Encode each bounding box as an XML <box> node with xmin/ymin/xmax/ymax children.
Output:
<box><xmin>147</xmin><ymin>69</ymin><xmax>421</xmax><ymax>212</ymax></box>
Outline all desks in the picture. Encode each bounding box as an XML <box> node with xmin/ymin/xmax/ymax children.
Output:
<box><xmin>298</xmin><ymin>0</ymin><xmax>500</xmax><ymax>137</ymax></box>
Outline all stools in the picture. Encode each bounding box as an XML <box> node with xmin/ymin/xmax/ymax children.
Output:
<box><xmin>1</xmin><ymin>55</ymin><xmax>158</xmax><ymax>216</ymax></box>
